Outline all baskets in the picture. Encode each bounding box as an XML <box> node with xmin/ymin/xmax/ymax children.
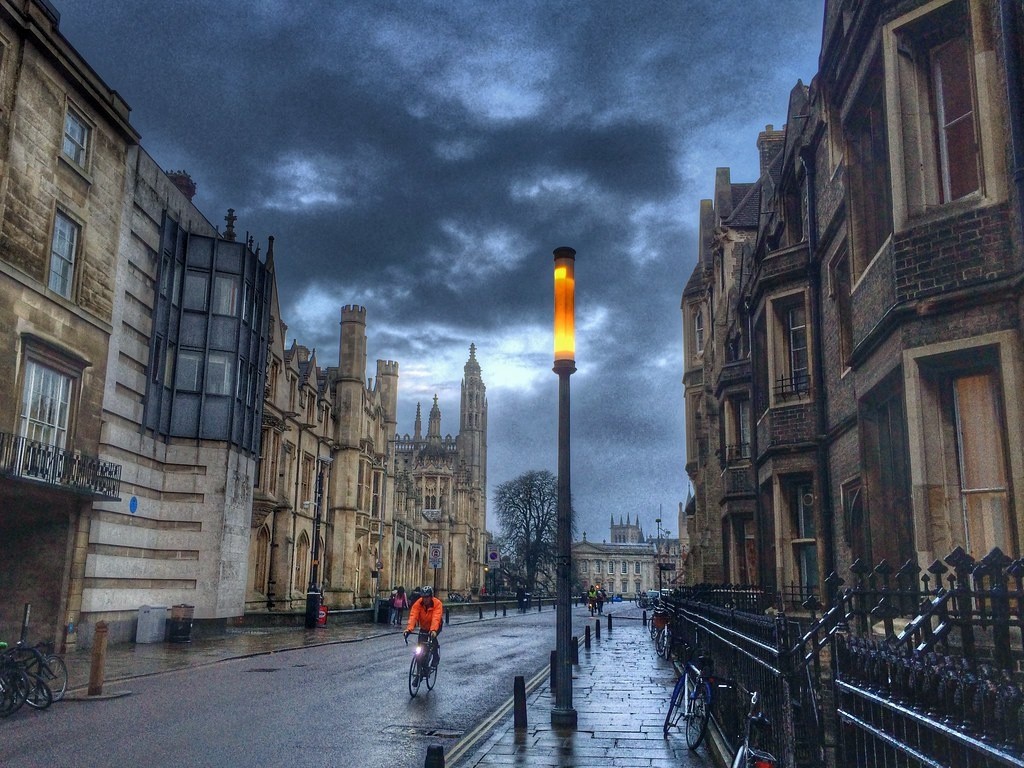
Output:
<box><xmin>653</xmin><ymin>614</ymin><xmax>665</xmax><ymax>628</ymax></box>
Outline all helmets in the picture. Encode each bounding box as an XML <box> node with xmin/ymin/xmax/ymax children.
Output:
<box><xmin>421</xmin><ymin>586</ymin><xmax>434</xmax><ymax>597</ymax></box>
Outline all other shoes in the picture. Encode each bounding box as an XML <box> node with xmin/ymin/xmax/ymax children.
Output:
<box><xmin>412</xmin><ymin>676</ymin><xmax>423</xmax><ymax>686</ymax></box>
<box><xmin>431</xmin><ymin>657</ymin><xmax>440</xmax><ymax>667</ymax></box>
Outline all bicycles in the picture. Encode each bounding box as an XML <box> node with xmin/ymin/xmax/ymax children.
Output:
<box><xmin>650</xmin><ymin>612</ymin><xmax>674</xmax><ymax>656</ymax></box>
<box><xmin>403</xmin><ymin>631</ymin><xmax>441</xmax><ymax>698</ymax></box>
<box><xmin>635</xmin><ymin>591</ymin><xmax>661</xmax><ymax>609</ymax></box>
<box><xmin>588</xmin><ymin>596</ymin><xmax>597</xmax><ymax>615</ymax></box>
<box><xmin>664</xmin><ymin>644</ymin><xmax>713</xmax><ymax>749</ymax></box>
<box><xmin>727</xmin><ymin>678</ymin><xmax>777</xmax><ymax>768</ymax></box>
<box><xmin>1</xmin><ymin>641</ymin><xmax>69</xmax><ymax>718</ymax></box>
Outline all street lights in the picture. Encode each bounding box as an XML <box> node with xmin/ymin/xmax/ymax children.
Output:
<box><xmin>302</xmin><ymin>455</ymin><xmax>333</xmax><ymax>629</ymax></box>
<box><xmin>552</xmin><ymin>246</ymin><xmax>580</xmax><ymax>725</ymax></box>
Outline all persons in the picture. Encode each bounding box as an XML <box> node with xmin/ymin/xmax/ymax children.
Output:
<box><xmin>390</xmin><ymin>586</ymin><xmax>399</xmax><ymax>625</ymax></box>
<box><xmin>482</xmin><ymin>584</ymin><xmax>485</xmax><ymax>595</ymax></box>
<box><xmin>516</xmin><ymin>586</ymin><xmax>525</xmax><ymax>612</ymax></box>
<box><xmin>587</xmin><ymin>585</ymin><xmax>597</xmax><ymax>610</ymax></box>
<box><xmin>393</xmin><ymin>586</ymin><xmax>409</xmax><ymax>626</ymax></box>
<box><xmin>409</xmin><ymin>586</ymin><xmax>421</xmax><ymax>610</ymax></box>
<box><xmin>405</xmin><ymin>585</ymin><xmax>443</xmax><ymax>687</ymax></box>
<box><xmin>448</xmin><ymin>593</ymin><xmax>472</xmax><ymax>603</ymax></box>
<box><xmin>597</xmin><ymin>588</ymin><xmax>606</xmax><ymax>615</ymax></box>
<box><xmin>636</xmin><ymin>589</ymin><xmax>653</xmax><ymax>599</ymax></box>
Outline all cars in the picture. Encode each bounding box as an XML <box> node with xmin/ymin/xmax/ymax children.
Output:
<box><xmin>661</xmin><ymin>588</ymin><xmax>673</xmax><ymax>596</ymax></box>
<box><xmin>647</xmin><ymin>590</ymin><xmax>660</xmax><ymax>602</ymax></box>
